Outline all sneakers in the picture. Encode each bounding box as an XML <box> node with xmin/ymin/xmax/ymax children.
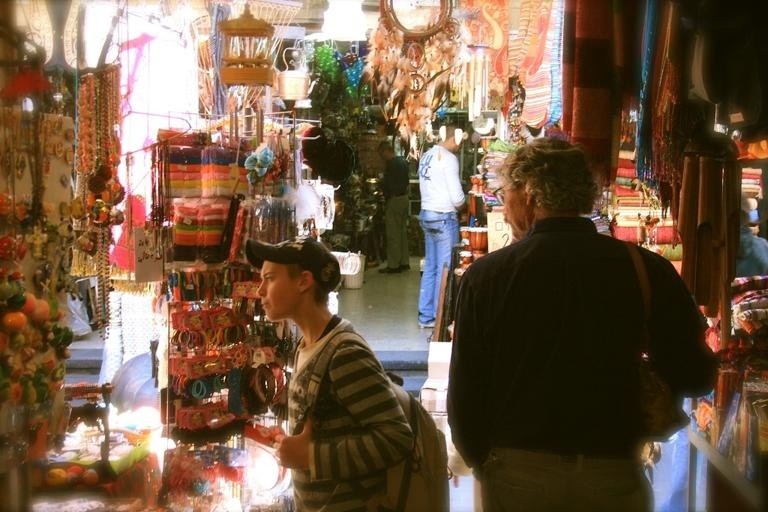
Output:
<box><xmin>417</xmin><ymin>316</ymin><xmax>437</xmax><ymax>331</ymax></box>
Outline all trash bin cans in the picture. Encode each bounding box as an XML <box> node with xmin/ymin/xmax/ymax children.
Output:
<box><xmin>343</xmin><ymin>254</ymin><xmax>366</xmax><ymax>288</ymax></box>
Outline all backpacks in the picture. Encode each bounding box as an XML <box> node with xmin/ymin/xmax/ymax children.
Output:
<box><xmin>364</xmin><ymin>374</ymin><xmax>451</xmax><ymax>512</ymax></box>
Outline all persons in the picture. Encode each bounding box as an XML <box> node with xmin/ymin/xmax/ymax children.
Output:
<box><xmin>378</xmin><ymin>142</ymin><xmax>411</xmax><ymax>273</ymax></box>
<box><xmin>246</xmin><ymin>238</ymin><xmax>414</xmax><ymax>512</ymax></box>
<box><xmin>417</xmin><ymin>123</ymin><xmax>470</xmax><ymax>327</ymax></box>
<box><xmin>447</xmin><ymin>137</ymin><xmax>717</xmax><ymax>512</ymax></box>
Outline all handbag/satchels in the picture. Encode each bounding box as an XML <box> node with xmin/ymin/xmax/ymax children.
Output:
<box><xmin>634</xmin><ymin>356</ymin><xmax>689</xmax><ymax>442</ymax></box>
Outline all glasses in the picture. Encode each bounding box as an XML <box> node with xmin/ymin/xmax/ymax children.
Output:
<box><xmin>492</xmin><ymin>179</ymin><xmax>517</xmax><ymax>205</ymax></box>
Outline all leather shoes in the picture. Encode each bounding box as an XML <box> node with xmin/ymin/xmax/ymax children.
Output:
<box><xmin>379</xmin><ymin>264</ymin><xmax>410</xmax><ymax>274</ymax></box>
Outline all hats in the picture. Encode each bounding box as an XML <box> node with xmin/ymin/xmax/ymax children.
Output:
<box><xmin>246</xmin><ymin>235</ymin><xmax>340</xmax><ymax>291</ymax></box>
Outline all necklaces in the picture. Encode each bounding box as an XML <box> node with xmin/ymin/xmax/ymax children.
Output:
<box><xmin>1</xmin><ymin>62</ymin><xmax>123</xmax><ymax>341</ymax></box>
